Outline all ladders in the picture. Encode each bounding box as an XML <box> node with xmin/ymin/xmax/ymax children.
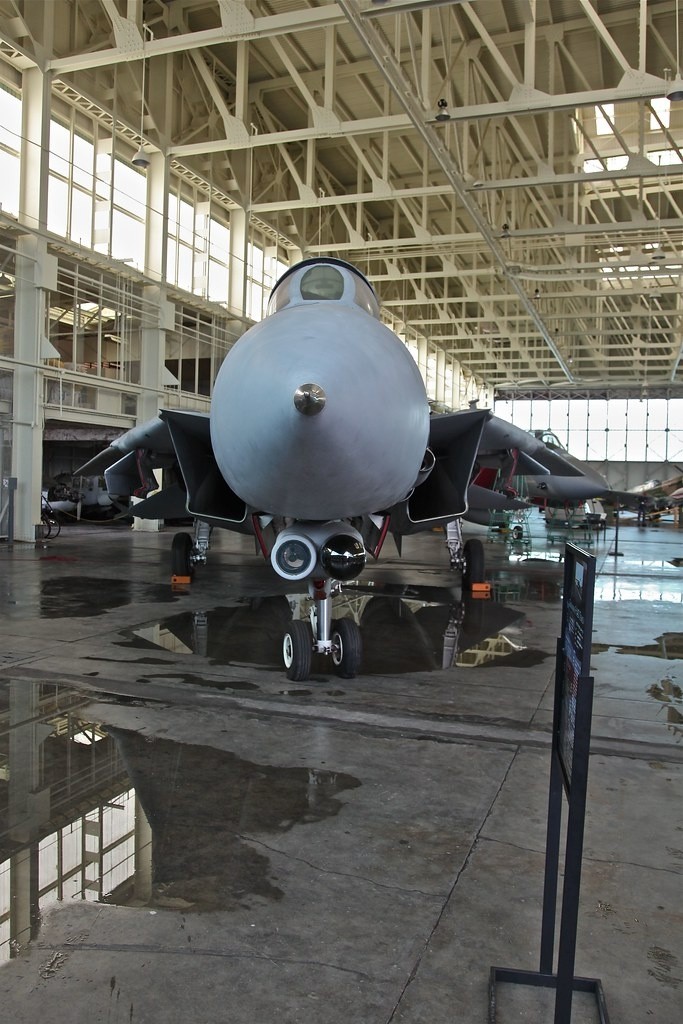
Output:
<box><xmin>545</xmin><ymin>498</ymin><xmax>596</xmax><ymax>547</ymax></box>
<box><xmin>485</xmin><ymin>467</ymin><xmax>533</xmax><ymax>546</ymax></box>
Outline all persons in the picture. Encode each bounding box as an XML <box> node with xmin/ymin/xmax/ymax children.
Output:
<box><xmin>637</xmin><ymin>496</ymin><xmax>648</xmax><ymax>527</ymax></box>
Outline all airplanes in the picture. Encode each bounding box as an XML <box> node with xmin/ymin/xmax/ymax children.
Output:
<box><xmin>495</xmin><ymin>430</ymin><xmax>648</xmax><ymax>510</ymax></box>
<box><xmin>74</xmin><ymin>258</ymin><xmax>584</xmax><ymax>683</ymax></box>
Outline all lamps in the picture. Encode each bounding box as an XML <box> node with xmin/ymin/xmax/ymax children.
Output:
<box><xmin>535</xmin><ymin>289</ymin><xmax>539</xmax><ymax>295</ymax></box>
<box><xmin>494</xmin><ymin>374</ymin><xmax>500</xmax><ymax>397</ymax></box>
<box><xmin>652</xmin><ymin>155</ymin><xmax>666</xmax><ymax>260</ymax></box>
<box><xmin>400</xmin><ymin>281</ymin><xmax>409</xmax><ymax>335</ymax></box>
<box><xmin>555</xmin><ymin>328</ymin><xmax>558</xmax><ymax>333</ymax></box>
<box><xmin>642</xmin><ymin>390</ymin><xmax>648</xmax><ymax>395</ymax></box>
<box><xmin>434</xmin><ymin>99</ymin><xmax>449</xmax><ymax>122</ymax></box>
<box><xmin>502</xmin><ymin>223</ymin><xmax>509</xmax><ymax>230</ymax></box>
<box><xmin>131</xmin><ymin>58</ymin><xmax>149</xmax><ymax>170</ymax></box>
<box><xmin>649</xmin><ymin>286</ymin><xmax>662</xmax><ymax>298</ymax></box>
<box><xmin>642</xmin><ymin>353</ymin><xmax>649</xmax><ymax>387</ymax></box>
